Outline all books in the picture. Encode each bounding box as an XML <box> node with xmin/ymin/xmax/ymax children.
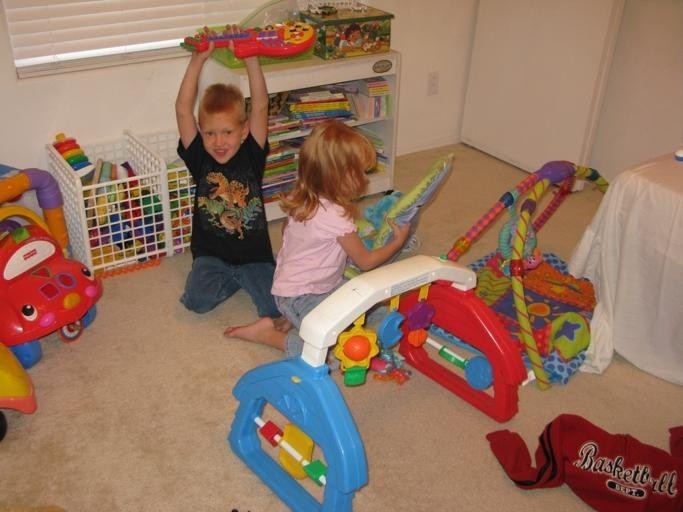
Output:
<box><xmin>243</xmin><ymin>78</ymin><xmax>393</xmax><ymax>202</ymax></box>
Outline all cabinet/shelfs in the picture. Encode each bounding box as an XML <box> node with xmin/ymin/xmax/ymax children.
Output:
<box><xmin>203</xmin><ymin>48</ymin><xmax>403</xmax><ymax>223</ymax></box>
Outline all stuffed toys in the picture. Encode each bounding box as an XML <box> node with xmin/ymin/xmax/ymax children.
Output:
<box><xmin>341</xmin><ymin>152</ymin><xmax>455</xmax><ymax>279</ymax></box>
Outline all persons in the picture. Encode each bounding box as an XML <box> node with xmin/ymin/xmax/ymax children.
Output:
<box><xmin>333</xmin><ymin>24</ymin><xmax>361</xmax><ymax>45</ymax></box>
<box><xmin>175</xmin><ymin>24</ymin><xmax>283</xmax><ymax>320</ymax></box>
<box><xmin>225</xmin><ymin>120</ymin><xmax>410</xmax><ymax>357</ymax></box>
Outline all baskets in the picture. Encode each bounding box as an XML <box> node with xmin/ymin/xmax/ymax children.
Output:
<box><xmin>47</xmin><ymin>131</ymin><xmax>197</xmax><ymax>274</ymax></box>
